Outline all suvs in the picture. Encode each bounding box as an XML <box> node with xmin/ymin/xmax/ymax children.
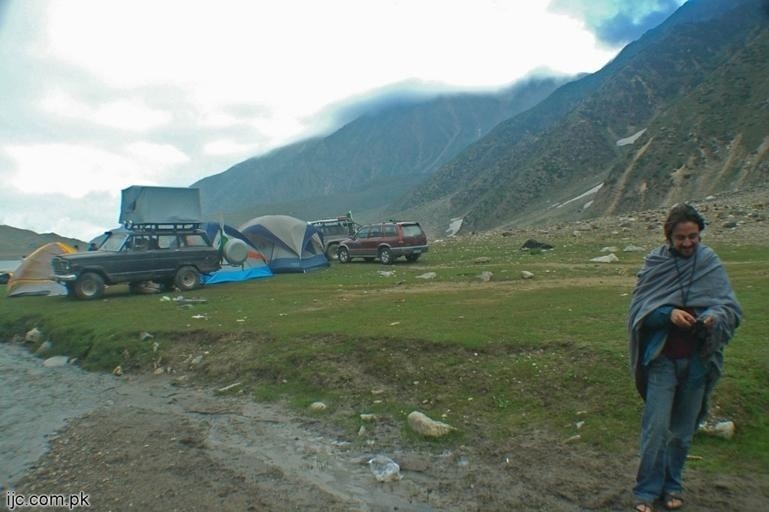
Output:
<box><xmin>50</xmin><ymin>220</ymin><xmax>224</xmax><ymax>299</ymax></box>
<box><xmin>306</xmin><ymin>217</ymin><xmax>356</xmax><ymax>260</ymax></box>
<box><xmin>338</xmin><ymin>219</ymin><xmax>429</xmax><ymax>265</ymax></box>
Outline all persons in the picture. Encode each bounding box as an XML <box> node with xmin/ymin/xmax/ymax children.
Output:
<box><xmin>624</xmin><ymin>204</ymin><xmax>744</xmax><ymax>511</ymax></box>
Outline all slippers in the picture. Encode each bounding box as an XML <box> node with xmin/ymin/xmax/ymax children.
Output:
<box><xmin>662</xmin><ymin>492</ymin><xmax>684</xmax><ymax>509</ymax></box>
<box><xmin>632</xmin><ymin>496</ymin><xmax>655</xmax><ymax>512</ymax></box>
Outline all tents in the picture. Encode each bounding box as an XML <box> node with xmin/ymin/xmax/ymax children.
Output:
<box><xmin>5</xmin><ymin>214</ymin><xmax>329</xmax><ymax>301</ymax></box>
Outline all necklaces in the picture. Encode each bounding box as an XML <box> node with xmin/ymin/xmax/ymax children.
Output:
<box><xmin>672</xmin><ymin>252</ymin><xmax>697</xmax><ymax>312</ymax></box>
